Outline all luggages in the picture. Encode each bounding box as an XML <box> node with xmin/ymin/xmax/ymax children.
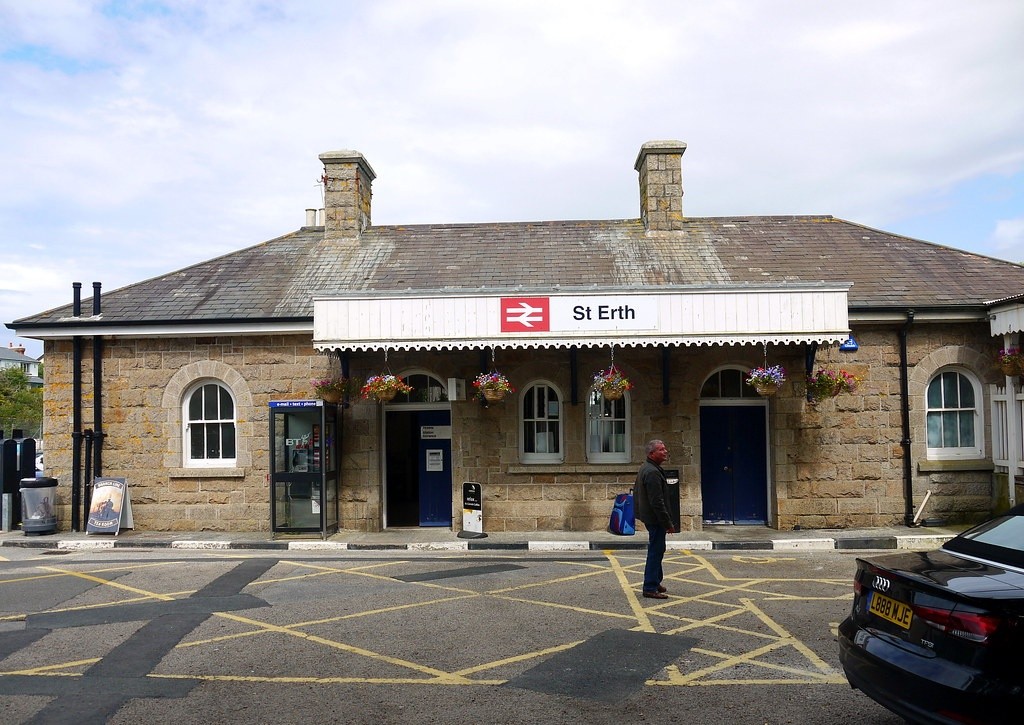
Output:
<box><xmin>610</xmin><ymin>488</ymin><xmax>636</xmax><ymax>536</ymax></box>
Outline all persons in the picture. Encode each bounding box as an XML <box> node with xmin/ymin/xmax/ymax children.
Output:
<box><xmin>632</xmin><ymin>439</ymin><xmax>675</xmax><ymax>599</ymax></box>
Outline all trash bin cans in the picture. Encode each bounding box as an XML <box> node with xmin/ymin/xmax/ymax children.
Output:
<box><xmin>19</xmin><ymin>477</ymin><xmax>58</xmax><ymax>536</ymax></box>
<box><xmin>664</xmin><ymin>469</ymin><xmax>680</xmax><ymax>533</ymax></box>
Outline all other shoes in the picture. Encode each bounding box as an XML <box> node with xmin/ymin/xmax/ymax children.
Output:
<box><xmin>643</xmin><ymin>585</ymin><xmax>667</xmax><ymax>593</ymax></box>
<box><xmin>643</xmin><ymin>589</ymin><xmax>668</xmax><ymax>599</ymax></box>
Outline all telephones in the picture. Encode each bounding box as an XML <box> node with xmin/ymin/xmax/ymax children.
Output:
<box><xmin>292</xmin><ymin>449</ymin><xmax>308</xmax><ymax>472</ymax></box>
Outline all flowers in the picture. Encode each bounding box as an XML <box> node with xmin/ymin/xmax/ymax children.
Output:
<box><xmin>745</xmin><ymin>364</ymin><xmax>789</xmax><ymax>387</ymax></box>
<box><xmin>591</xmin><ymin>362</ymin><xmax>637</xmax><ymax>395</ymax></box>
<box><xmin>470</xmin><ymin>371</ymin><xmax>516</xmax><ymax>402</ymax></box>
<box><xmin>805</xmin><ymin>368</ymin><xmax>857</xmax><ymax>403</ymax></box>
<box><xmin>309</xmin><ymin>372</ymin><xmax>359</xmax><ymax>399</ymax></box>
<box><xmin>360</xmin><ymin>374</ymin><xmax>415</xmax><ymax>399</ymax></box>
<box><xmin>996</xmin><ymin>345</ymin><xmax>1024</xmax><ymax>369</ymax></box>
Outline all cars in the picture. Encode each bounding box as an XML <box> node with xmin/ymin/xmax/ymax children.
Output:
<box><xmin>837</xmin><ymin>506</ymin><xmax>1023</xmax><ymax>725</ymax></box>
